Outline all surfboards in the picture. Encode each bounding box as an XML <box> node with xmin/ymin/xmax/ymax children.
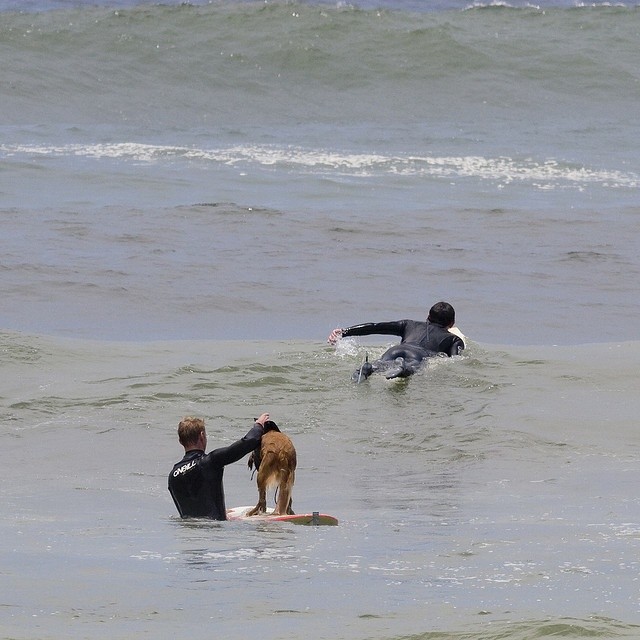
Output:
<box><xmin>447</xmin><ymin>327</ymin><xmax>466</xmax><ymax>349</ymax></box>
<box><xmin>226</xmin><ymin>506</ymin><xmax>338</xmax><ymax>527</ymax></box>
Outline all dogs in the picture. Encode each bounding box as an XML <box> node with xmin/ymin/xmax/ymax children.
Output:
<box><xmin>247</xmin><ymin>430</ymin><xmax>296</xmax><ymax>516</ymax></box>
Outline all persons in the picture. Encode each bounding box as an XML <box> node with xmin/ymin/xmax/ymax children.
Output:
<box><xmin>326</xmin><ymin>301</ymin><xmax>464</xmax><ymax>385</ymax></box>
<box><xmin>167</xmin><ymin>412</ymin><xmax>270</xmax><ymax>521</ymax></box>
